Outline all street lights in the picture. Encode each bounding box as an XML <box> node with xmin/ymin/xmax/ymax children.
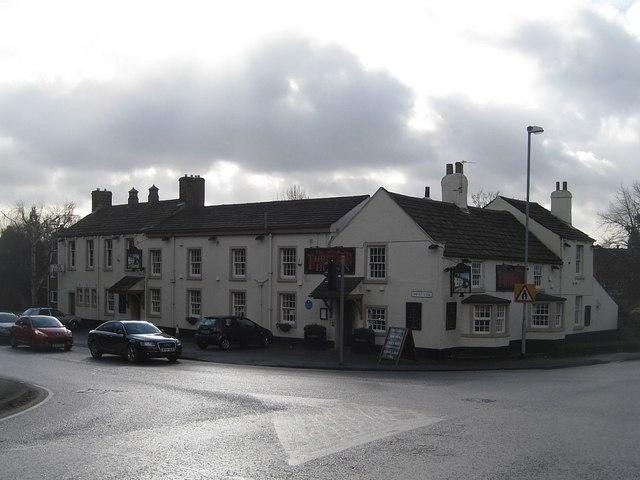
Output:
<box><xmin>521</xmin><ymin>122</ymin><xmax>545</xmax><ymax>356</ymax></box>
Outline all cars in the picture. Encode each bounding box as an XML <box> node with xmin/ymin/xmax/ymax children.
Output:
<box><xmin>20</xmin><ymin>307</ymin><xmax>82</xmax><ymax>329</ymax></box>
<box><xmin>10</xmin><ymin>314</ymin><xmax>75</xmax><ymax>352</ymax></box>
<box><xmin>87</xmin><ymin>320</ymin><xmax>184</xmax><ymax>365</ymax></box>
<box><xmin>0</xmin><ymin>311</ymin><xmax>21</xmax><ymax>341</ymax></box>
<box><xmin>194</xmin><ymin>315</ymin><xmax>274</xmax><ymax>350</ymax></box>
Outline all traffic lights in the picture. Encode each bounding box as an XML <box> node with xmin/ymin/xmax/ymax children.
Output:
<box><xmin>324</xmin><ymin>261</ymin><xmax>332</xmax><ymax>291</ymax></box>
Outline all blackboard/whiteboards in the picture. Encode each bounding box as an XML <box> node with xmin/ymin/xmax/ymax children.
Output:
<box><xmin>379</xmin><ymin>326</ymin><xmax>408</xmax><ymax>362</ymax></box>
